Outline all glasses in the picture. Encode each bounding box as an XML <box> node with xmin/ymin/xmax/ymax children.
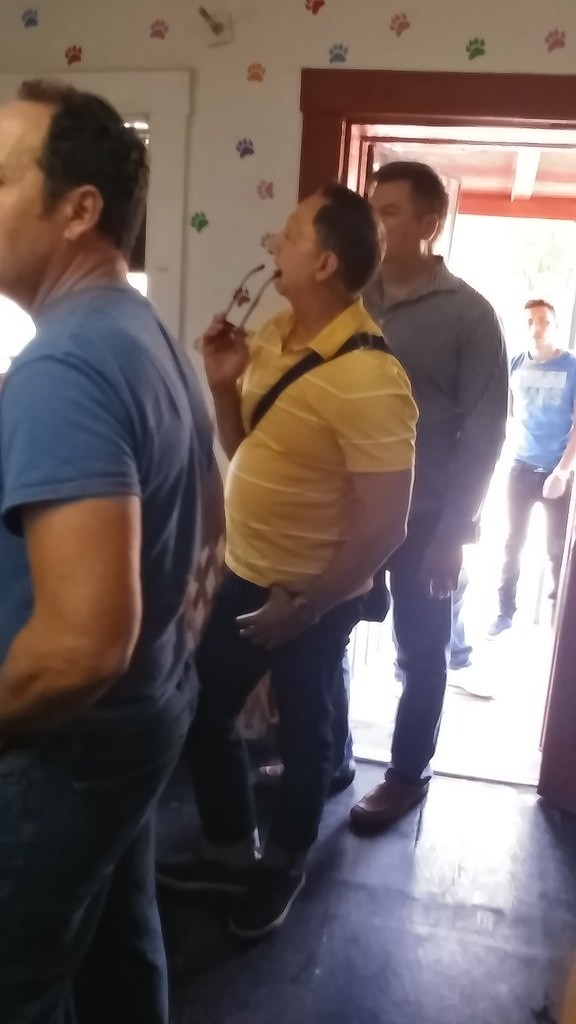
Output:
<box><xmin>213</xmin><ymin>264</ymin><xmax>283</xmax><ymax>337</ymax></box>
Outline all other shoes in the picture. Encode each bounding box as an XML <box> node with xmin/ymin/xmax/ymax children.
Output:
<box><xmin>444</xmin><ymin>662</ymin><xmax>495</xmax><ymax>700</ymax></box>
<box><xmin>155</xmin><ymin>845</ymin><xmax>259</xmax><ymax>893</ymax></box>
<box><xmin>228</xmin><ymin>867</ymin><xmax>308</xmax><ymax>940</ymax></box>
<box><xmin>488</xmin><ymin>613</ymin><xmax>512</xmax><ymax>635</ymax></box>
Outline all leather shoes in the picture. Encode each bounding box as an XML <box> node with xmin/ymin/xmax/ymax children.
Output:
<box><xmin>350</xmin><ymin>776</ymin><xmax>433</xmax><ymax>829</ymax></box>
<box><xmin>252</xmin><ymin>765</ymin><xmax>356</xmax><ymax>797</ymax></box>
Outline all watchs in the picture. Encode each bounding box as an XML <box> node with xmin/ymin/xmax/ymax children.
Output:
<box><xmin>553</xmin><ymin>467</ymin><xmax>570</xmax><ymax>480</ymax></box>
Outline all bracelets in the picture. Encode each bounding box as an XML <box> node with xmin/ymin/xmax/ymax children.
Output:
<box><xmin>294</xmin><ymin>595</ymin><xmax>320</xmax><ymax>626</ymax></box>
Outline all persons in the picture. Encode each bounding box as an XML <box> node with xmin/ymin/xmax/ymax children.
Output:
<box><xmin>486</xmin><ymin>299</ymin><xmax>576</xmax><ymax>640</ymax></box>
<box><xmin>155</xmin><ymin>181</ymin><xmax>418</xmax><ymax>939</ymax></box>
<box><xmin>260</xmin><ymin>160</ymin><xmax>508</xmax><ymax>831</ymax></box>
<box><xmin>0</xmin><ymin>79</ymin><xmax>227</xmax><ymax>1024</ymax></box>
<box><xmin>393</xmin><ymin>567</ymin><xmax>495</xmax><ymax>701</ymax></box>
